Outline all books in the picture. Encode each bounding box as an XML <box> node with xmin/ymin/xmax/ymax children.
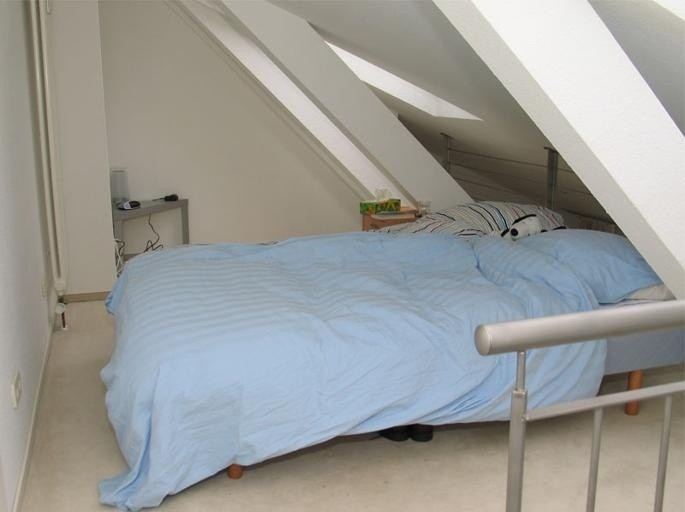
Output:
<box><xmin>371</xmin><ymin>207</ymin><xmax>418</xmax><ymax>220</ymax></box>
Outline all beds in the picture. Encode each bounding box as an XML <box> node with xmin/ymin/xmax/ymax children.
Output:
<box><xmin>111</xmin><ymin>198</ymin><xmax>680</xmax><ymax>478</ymax></box>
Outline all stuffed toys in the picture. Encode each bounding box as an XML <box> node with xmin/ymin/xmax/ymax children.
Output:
<box><xmin>501</xmin><ymin>214</ymin><xmax>548</xmax><ymax>241</ymax></box>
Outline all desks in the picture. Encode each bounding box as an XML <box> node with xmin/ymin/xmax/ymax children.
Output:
<box><xmin>113</xmin><ymin>197</ymin><xmax>189</xmax><ymax>264</ymax></box>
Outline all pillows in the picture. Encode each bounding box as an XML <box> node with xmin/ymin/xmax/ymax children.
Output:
<box><xmin>379</xmin><ymin>202</ymin><xmax>658</xmax><ymax>305</ymax></box>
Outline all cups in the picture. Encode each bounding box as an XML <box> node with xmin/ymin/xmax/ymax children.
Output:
<box><xmin>417</xmin><ymin>199</ymin><xmax>431</xmax><ymax>216</ymax></box>
